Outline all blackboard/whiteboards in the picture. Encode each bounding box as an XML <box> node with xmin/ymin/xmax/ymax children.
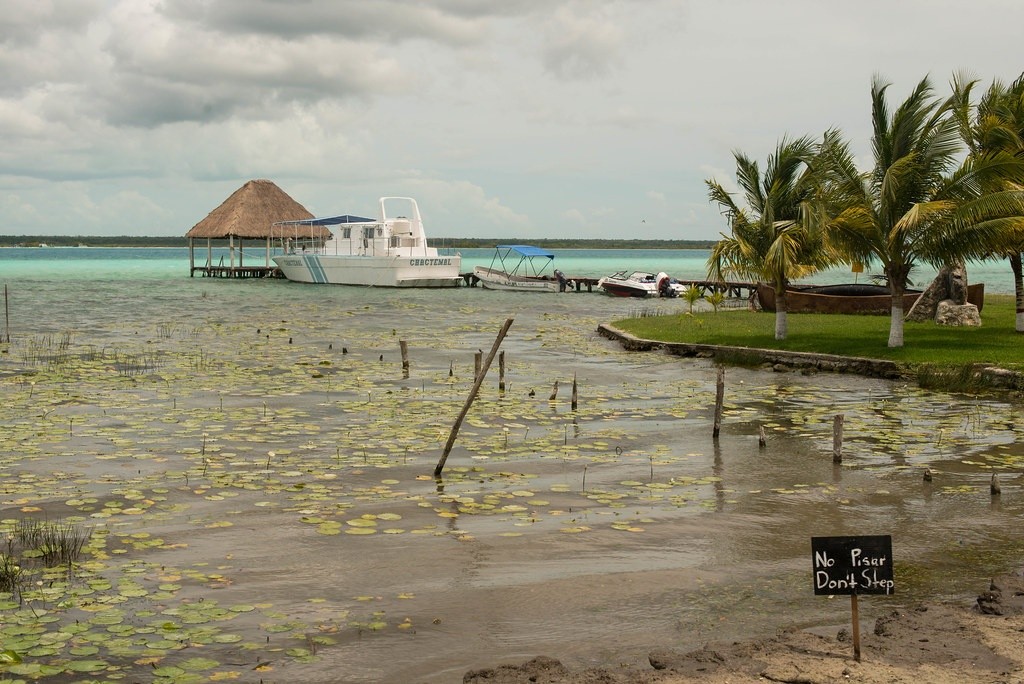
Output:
<box><xmin>810</xmin><ymin>535</ymin><xmax>894</xmax><ymax>596</ymax></box>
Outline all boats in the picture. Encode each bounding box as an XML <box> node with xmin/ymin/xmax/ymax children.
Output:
<box><xmin>473</xmin><ymin>244</ymin><xmax>576</xmax><ymax>293</ymax></box>
<box><xmin>756</xmin><ymin>280</ymin><xmax>985</xmax><ymax>318</ymax></box>
<box><xmin>597</xmin><ymin>270</ymin><xmax>688</xmax><ymax>299</ymax></box>
<box><xmin>270</xmin><ymin>196</ymin><xmax>465</xmax><ymax>289</ymax></box>
